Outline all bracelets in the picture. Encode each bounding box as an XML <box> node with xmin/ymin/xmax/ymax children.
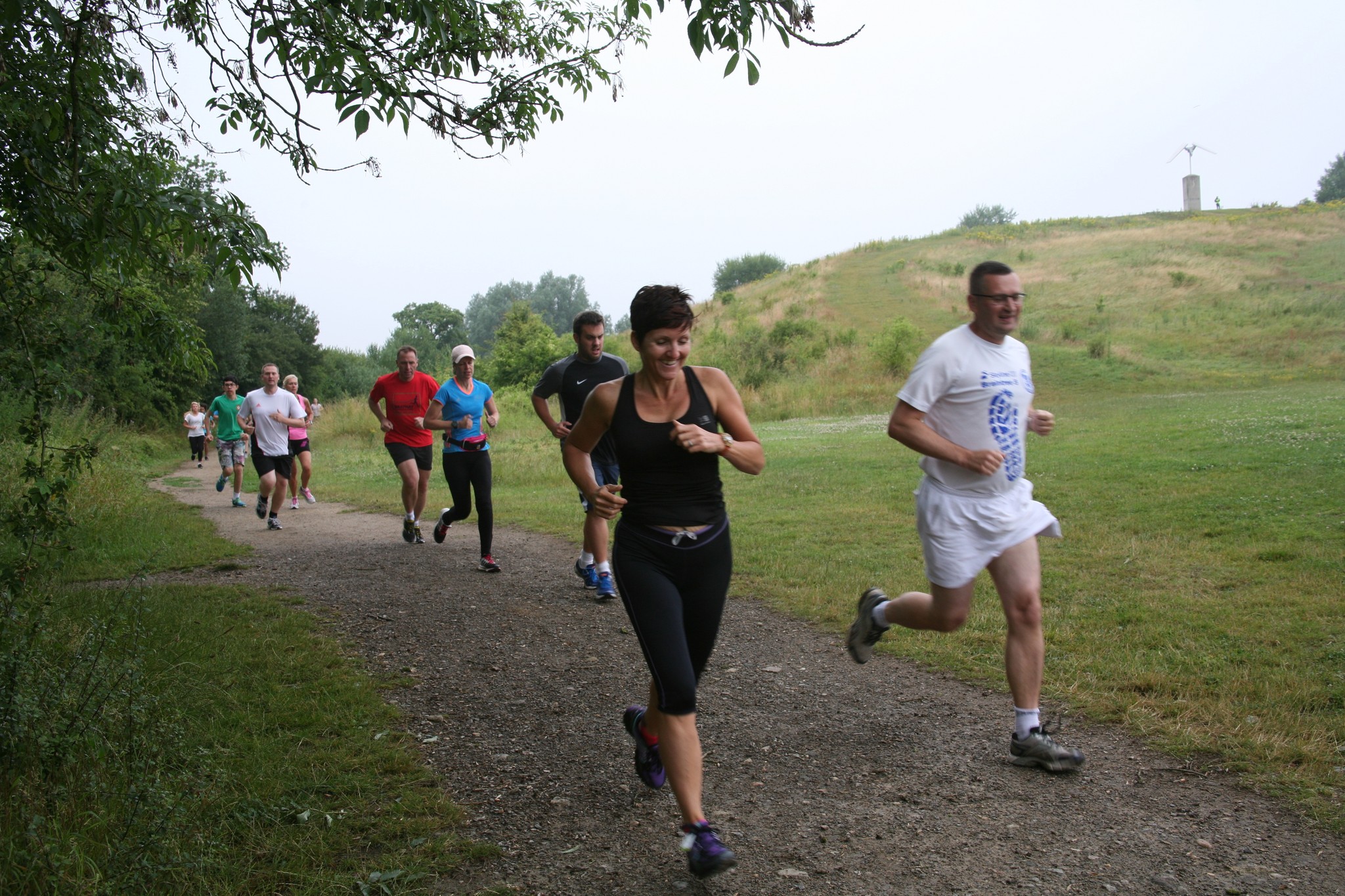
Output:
<box><xmin>1027</xmin><ymin>410</ymin><xmax>1036</xmax><ymax>430</ymax></box>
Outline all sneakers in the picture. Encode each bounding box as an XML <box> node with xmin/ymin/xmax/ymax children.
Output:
<box><xmin>232</xmin><ymin>498</ymin><xmax>246</xmax><ymax>508</ymax></box>
<box><xmin>191</xmin><ymin>454</ymin><xmax>196</xmax><ymax>460</ymax></box>
<box><xmin>680</xmin><ymin>821</ymin><xmax>735</xmax><ymax>879</ymax></box>
<box><xmin>847</xmin><ymin>588</ymin><xmax>891</xmax><ymax>664</ymax></box>
<box><xmin>216</xmin><ymin>473</ymin><xmax>229</xmax><ymax>492</ymax></box>
<box><xmin>299</xmin><ymin>487</ymin><xmax>316</xmax><ymax>504</ymax></box>
<box><xmin>597</xmin><ymin>572</ymin><xmax>617</xmax><ymax>600</ymax></box>
<box><xmin>267</xmin><ymin>517</ymin><xmax>283</xmax><ymax>530</ymax></box>
<box><xmin>414</xmin><ymin>526</ymin><xmax>426</xmax><ymax>544</ymax></box>
<box><xmin>256</xmin><ymin>493</ymin><xmax>268</xmax><ymax>518</ymax></box>
<box><xmin>1009</xmin><ymin>715</ymin><xmax>1085</xmax><ymax>771</ymax></box>
<box><xmin>478</xmin><ymin>554</ymin><xmax>502</xmax><ymax>571</ymax></box>
<box><xmin>198</xmin><ymin>464</ymin><xmax>203</xmax><ymax>469</ymax></box>
<box><xmin>623</xmin><ymin>705</ymin><xmax>666</xmax><ymax>789</ymax></box>
<box><xmin>402</xmin><ymin>516</ymin><xmax>417</xmax><ymax>544</ymax></box>
<box><xmin>574</xmin><ymin>558</ymin><xmax>599</xmax><ymax>589</ymax></box>
<box><xmin>433</xmin><ymin>506</ymin><xmax>452</xmax><ymax>544</ymax></box>
<box><xmin>289</xmin><ymin>496</ymin><xmax>299</xmax><ymax>509</ymax></box>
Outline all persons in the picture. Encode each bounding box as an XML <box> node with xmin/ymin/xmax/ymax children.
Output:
<box><xmin>846</xmin><ymin>259</ymin><xmax>1083</xmax><ymax>769</ymax></box>
<box><xmin>567</xmin><ymin>285</ymin><xmax>767</xmax><ymax>879</ymax></box>
<box><xmin>183</xmin><ymin>308</ymin><xmax>644</xmax><ymax>600</ymax></box>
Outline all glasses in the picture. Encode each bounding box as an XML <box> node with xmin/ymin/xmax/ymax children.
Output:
<box><xmin>972</xmin><ymin>294</ymin><xmax>1026</xmax><ymax>306</ymax></box>
<box><xmin>224</xmin><ymin>383</ymin><xmax>236</xmax><ymax>387</ymax></box>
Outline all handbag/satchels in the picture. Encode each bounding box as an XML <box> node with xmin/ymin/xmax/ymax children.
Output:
<box><xmin>442</xmin><ymin>431</ymin><xmax>487</xmax><ymax>452</ymax></box>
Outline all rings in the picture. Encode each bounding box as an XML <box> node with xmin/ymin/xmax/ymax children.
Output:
<box><xmin>274</xmin><ymin>417</ymin><xmax>276</xmax><ymax>419</ymax></box>
<box><xmin>688</xmin><ymin>441</ymin><xmax>691</xmax><ymax>448</ymax></box>
<box><xmin>491</xmin><ymin>421</ymin><xmax>494</xmax><ymax>424</ymax></box>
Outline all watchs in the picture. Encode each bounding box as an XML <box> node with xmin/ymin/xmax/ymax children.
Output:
<box><xmin>452</xmin><ymin>419</ymin><xmax>456</xmax><ymax>428</ymax></box>
<box><xmin>717</xmin><ymin>430</ymin><xmax>733</xmax><ymax>455</ymax></box>
<box><xmin>308</xmin><ymin>420</ymin><xmax>313</xmax><ymax>425</ymax></box>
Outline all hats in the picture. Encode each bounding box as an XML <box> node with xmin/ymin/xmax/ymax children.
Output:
<box><xmin>451</xmin><ymin>344</ymin><xmax>475</xmax><ymax>363</ymax></box>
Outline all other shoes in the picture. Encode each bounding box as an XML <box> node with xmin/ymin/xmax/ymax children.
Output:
<box><xmin>243</xmin><ymin>452</ymin><xmax>247</xmax><ymax>458</ymax></box>
<box><xmin>205</xmin><ymin>457</ymin><xmax>209</xmax><ymax>461</ymax></box>
